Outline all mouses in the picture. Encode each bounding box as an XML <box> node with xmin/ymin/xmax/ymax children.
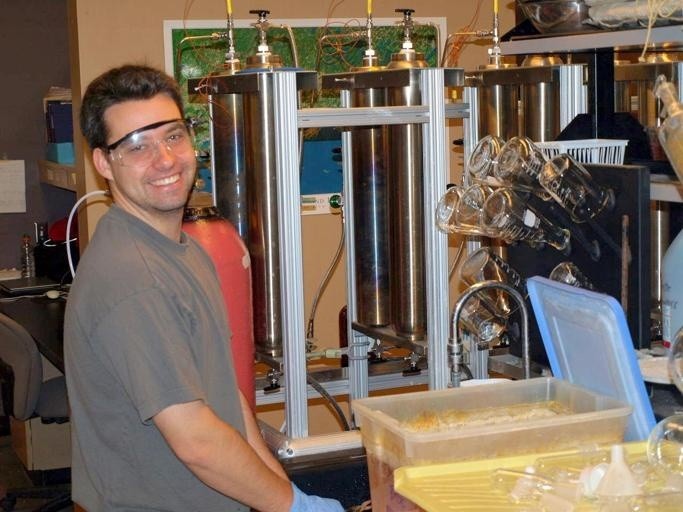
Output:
<box><xmin>45</xmin><ymin>290</ymin><xmax>60</xmax><ymax>300</ymax></box>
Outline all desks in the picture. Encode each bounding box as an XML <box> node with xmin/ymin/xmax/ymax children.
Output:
<box><xmin>0</xmin><ymin>284</ymin><xmax>71</xmax><ymax>471</ymax></box>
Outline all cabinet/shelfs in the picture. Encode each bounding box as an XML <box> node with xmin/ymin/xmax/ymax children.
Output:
<box><xmin>488</xmin><ymin>25</ymin><xmax>683</xmax><ymax>387</ymax></box>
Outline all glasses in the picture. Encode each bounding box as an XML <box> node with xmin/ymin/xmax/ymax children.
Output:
<box><xmin>102</xmin><ymin>118</ymin><xmax>198</xmax><ymax>166</ymax></box>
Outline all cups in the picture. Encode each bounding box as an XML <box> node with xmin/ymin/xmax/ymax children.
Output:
<box><xmin>458</xmin><ymin>246</ymin><xmax>531</xmax><ymax>318</ymax></box>
<box><xmin>537</xmin><ymin>152</ymin><xmax>609</xmax><ymax>224</ymax></box>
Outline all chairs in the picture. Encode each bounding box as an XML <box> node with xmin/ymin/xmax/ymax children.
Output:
<box><xmin>0</xmin><ymin>311</ymin><xmax>73</xmax><ymax>512</ymax></box>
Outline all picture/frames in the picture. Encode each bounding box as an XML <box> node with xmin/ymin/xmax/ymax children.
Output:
<box><xmin>161</xmin><ymin>15</ymin><xmax>449</xmax><ymax>216</ymax></box>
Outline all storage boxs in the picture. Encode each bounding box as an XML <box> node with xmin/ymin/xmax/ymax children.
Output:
<box><xmin>350</xmin><ymin>377</ymin><xmax>632</xmax><ymax>512</ymax></box>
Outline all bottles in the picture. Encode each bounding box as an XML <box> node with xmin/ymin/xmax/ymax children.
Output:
<box><xmin>20</xmin><ymin>233</ymin><xmax>34</xmax><ymax>278</ymax></box>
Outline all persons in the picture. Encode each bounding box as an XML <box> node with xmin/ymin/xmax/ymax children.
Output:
<box><xmin>65</xmin><ymin>65</ymin><xmax>350</xmax><ymax>512</ymax></box>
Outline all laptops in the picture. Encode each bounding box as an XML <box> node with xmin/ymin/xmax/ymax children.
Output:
<box><xmin>0</xmin><ymin>276</ymin><xmax>61</xmax><ymax>294</ymax></box>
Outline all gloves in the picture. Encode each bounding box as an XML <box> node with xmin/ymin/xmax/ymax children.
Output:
<box><xmin>291</xmin><ymin>482</ymin><xmax>345</xmax><ymax>512</ymax></box>
